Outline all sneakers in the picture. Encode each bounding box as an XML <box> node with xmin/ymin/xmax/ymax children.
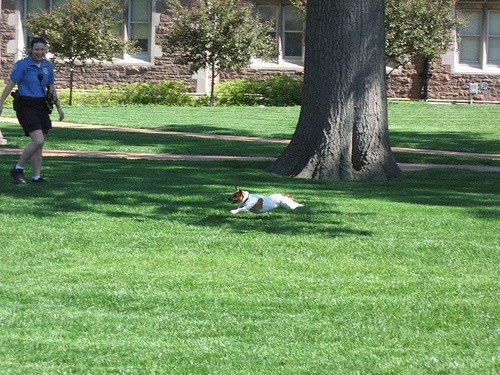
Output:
<box><xmin>10</xmin><ymin>165</ymin><xmax>27</xmax><ymax>184</ymax></box>
<box><xmin>32</xmin><ymin>177</ymin><xmax>48</xmax><ymax>183</ymax></box>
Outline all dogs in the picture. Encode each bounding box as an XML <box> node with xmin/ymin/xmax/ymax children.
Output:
<box><xmin>228</xmin><ymin>185</ymin><xmax>305</xmax><ymax>215</ymax></box>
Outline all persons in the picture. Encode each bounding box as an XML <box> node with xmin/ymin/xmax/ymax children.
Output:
<box><xmin>0</xmin><ymin>37</ymin><xmax>64</xmax><ymax>184</ymax></box>
